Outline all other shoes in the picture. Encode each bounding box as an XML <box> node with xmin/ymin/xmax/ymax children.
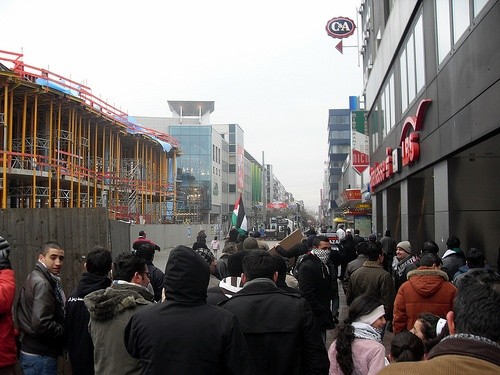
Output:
<box><xmin>334</xmin><ymin>315</ymin><xmax>339</xmax><ymax>324</ymax></box>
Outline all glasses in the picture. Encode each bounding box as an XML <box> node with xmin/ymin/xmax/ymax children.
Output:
<box><xmin>144</xmin><ymin>272</ymin><xmax>152</xmax><ymax>278</ymax></box>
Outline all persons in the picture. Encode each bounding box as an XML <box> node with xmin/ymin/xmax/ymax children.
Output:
<box><xmin>124</xmin><ymin>245</ymin><xmax>254</xmax><ymax>375</ymax></box>
<box><xmin>388</xmin><ymin>331</ymin><xmax>424</xmax><ymax>364</ymax></box>
<box><xmin>328</xmin><ymin>295</ymin><xmax>387</xmax><ymax>375</ymax></box>
<box><xmin>63</xmin><ymin>246</ymin><xmax>113</xmax><ymax>375</ymax></box>
<box><xmin>0</xmin><ymin>235</ymin><xmax>18</xmax><ymax>375</ymax></box>
<box><xmin>409</xmin><ymin>312</ymin><xmax>450</xmax><ymax>362</ymax></box>
<box><xmin>295</xmin><ymin>235</ymin><xmax>340</xmax><ymax>345</ymax></box>
<box><xmin>391</xmin><ymin>251</ymin><xmax>458</xmax><ymax>334</ymax></box>
<box><xmin>16</xmin><ymin>241</ymin><xmax>67</xmax><ymax>375</ymax></box>
<box><xmin>85</xmin><ymin>250</ymin><xmax>157</xmax><ymax>375</ymax></box>
<box><xmin>206</xmin><ymin>250</ymin><xmax>246</xmax><ymax>307</ymax></box>
<box><xmin>346</xmin><ymin>244</ymin><xmax>397</xmax><ymax>321</ymax></box>
<box><xmin>125</xmin><ymin>213</ymin><xmax>500</xmax><ymax>289</ymax></box>
<box><xmin>377</xmin><ymin>268</ymin><xmax>500</xmax><ymax>375</ymax></box>
<box><xmin>136</xmin><ymin>244</ymin><xmax>165</xmax><ymax>304</ymax></box>
<box><xmin>217</xmin><ymin>250</ymin><xmax>331</xmax><ymax>375</ymax></box>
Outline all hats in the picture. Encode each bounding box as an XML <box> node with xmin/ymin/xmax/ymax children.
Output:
<box><xmin>396</xmin><ymin>241</ymin><xmax>412</xmax><ymax>254</ymax></box>
<box><xmin>346</xmin><ymin>228</ymin><xmax>351</xmax><ymax>233</ymax></box>
<box><xmin>195</xmin><ymin>248</ymin><xmax>215</xmax><ymax>265</ymax></box>
<box><xmin>0</xmin><ymin>236</ymin><xmax>11</xmax><ymax>258</ymax></box>
